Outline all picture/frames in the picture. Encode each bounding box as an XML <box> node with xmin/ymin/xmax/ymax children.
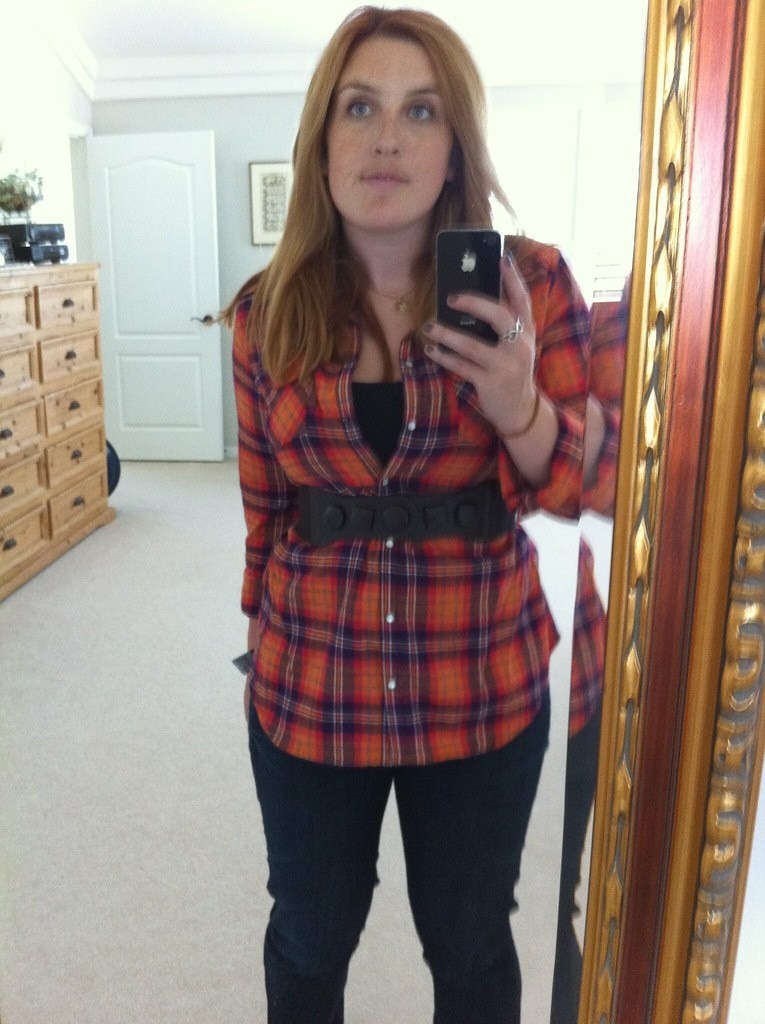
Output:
<box><xmin>249</xmin><ymin>160</ymin><xmax>295</xmax><ymax>246</ymax></box>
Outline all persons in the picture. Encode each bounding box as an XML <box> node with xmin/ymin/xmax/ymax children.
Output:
<box><xmin>549</xmin><ymin>302</ymin><xmax>631</xmax><ymax>1024</ymax></box>
<box><xmin>193</xmin><ymin>5</ymin><xmax>591</xmax><ymax>1024</ymax></box>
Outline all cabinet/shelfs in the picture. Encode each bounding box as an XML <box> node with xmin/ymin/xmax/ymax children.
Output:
<box><xmin>0</xmin><ymin>258</ymin><xmax>118</xmax><ymax>601</ymax></box>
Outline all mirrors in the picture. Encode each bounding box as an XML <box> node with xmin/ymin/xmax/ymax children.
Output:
<box><xmin>0</xmin><ymin>0</ymin><xmax>765</xmax><ymax>1024</ymax></box>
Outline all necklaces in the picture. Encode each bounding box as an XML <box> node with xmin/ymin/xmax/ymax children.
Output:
<box><xmin>368</xmin><ymin>285</ymin><xmax>416</xmax><ymax>312</ymax></box>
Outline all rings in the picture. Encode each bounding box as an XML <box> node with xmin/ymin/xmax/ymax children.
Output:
<box><xmin>501</xmin><ymin>316</ymin><xmax>525</xmax><ymax>347</ymax></box>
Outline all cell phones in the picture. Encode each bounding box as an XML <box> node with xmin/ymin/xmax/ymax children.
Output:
<box><xmin>435</xmin><ymin>229</ymin><xmax>502</xmax><ymax>353</ymax></box>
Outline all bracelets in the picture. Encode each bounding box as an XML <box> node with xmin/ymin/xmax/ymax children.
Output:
<box><xmin>503</xmin><ymin>393</ymin><xmax>542</xmax><ymax>440</ymax></box>
